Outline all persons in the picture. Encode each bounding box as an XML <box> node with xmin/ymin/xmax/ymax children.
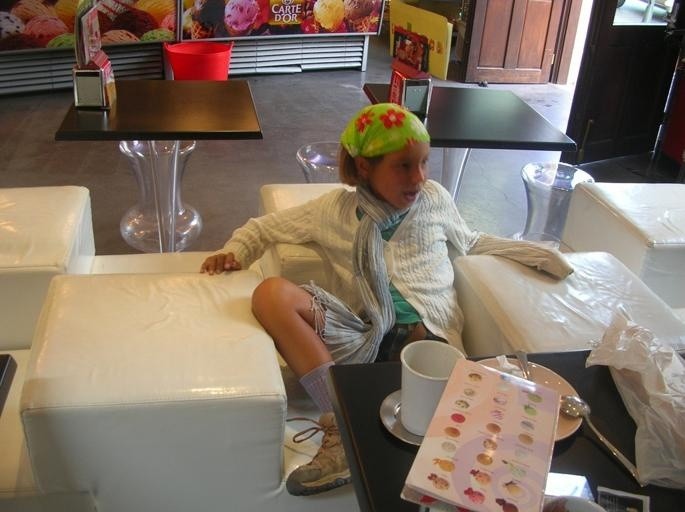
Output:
<box><xmin>199</xmin><ymin>102</ymin><xmax>575</xmax><ymax>496</ymax></box>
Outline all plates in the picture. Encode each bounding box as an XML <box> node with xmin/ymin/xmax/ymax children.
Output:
<box><xmin>473</xmin><ymin>356</ymin><xmax>584</xmax><ymax>444</ymax></box>
<box><xmin>380</xmin><ymin>388</ymin><xmax>424</xmax><ymax>446</ymax></box>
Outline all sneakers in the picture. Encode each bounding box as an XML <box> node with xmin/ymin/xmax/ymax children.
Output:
<box><xmin>284</xmin><ymin>411</ymin><xmax>354</xmax><ymax>497</ymax></box>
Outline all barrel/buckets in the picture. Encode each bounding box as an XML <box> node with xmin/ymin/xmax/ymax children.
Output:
<box><xmin>162</xmin><ymin>40</ymin><xmax>236</xmax><ymax>81</ymax></box>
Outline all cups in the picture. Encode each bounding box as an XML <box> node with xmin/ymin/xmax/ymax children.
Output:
<box><xmin>398</xmin><ymin>340</ymin><xmax>466</xmax><ymax>437</ymax></box>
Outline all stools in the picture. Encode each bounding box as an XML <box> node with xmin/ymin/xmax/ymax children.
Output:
<box><xmin>18</xmin><ymin>267</ymin><xmax>288</xmax><ymax>512</ymax></box>
<box><xmin>254</xmin><ymin>185</ymin><xmax>356</xmax><ymax>289</ymax></box>
<box><xmin>557</xmin><ymin>182</ymin><xmax>685</xmax><ymax>307</ymax></box>
<box><xmin>451</xmin><ymin>250</ymin><xmax>685</xmax><ymax>355</ymax></box>
<box><xmin>0</xmin><ymin>185</ymin><xmax>97</xmax><ymax>352</ymax></box>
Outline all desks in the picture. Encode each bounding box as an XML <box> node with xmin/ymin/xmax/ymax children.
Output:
<box><xmin>363</xmin><ymin>82</ymin><xmax>578</xmax><ymax>203</ymax></box>
<box><xmin>54</xmin><ymin>80</ymin><xmax>264</xmax><ymax>254</ymax></box>
<box><xmin>325</xmin><ymin>349</ymin><xmax>685</xmax><ymax>511</ymax></box>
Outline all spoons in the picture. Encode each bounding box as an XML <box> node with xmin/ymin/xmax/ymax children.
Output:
<box><xmin>559</xmin><ymin>396</ymin><xmax>652</xmax><ymax>490</ymax></box>
